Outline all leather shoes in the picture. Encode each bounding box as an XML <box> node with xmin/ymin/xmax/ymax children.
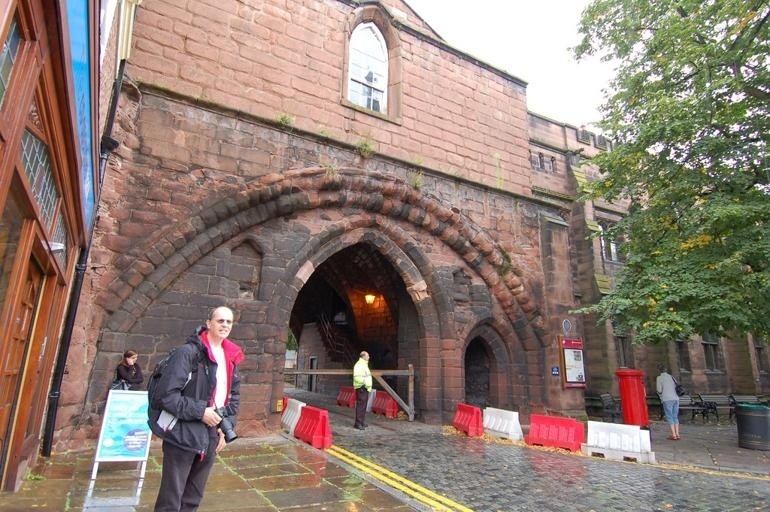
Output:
<box><xmin>354</xmin><ymin>423</ymin><xmax>368</xmax><ymax>430</ymax></box>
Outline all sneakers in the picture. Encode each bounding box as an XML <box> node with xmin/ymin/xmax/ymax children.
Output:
<box><xmin>670</xmin><ymin>435</ymin><xmax>680</xmax><ymax>439</ymax></box>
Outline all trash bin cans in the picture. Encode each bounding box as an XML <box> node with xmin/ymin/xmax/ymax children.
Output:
<box><xmin>735</xmin><ymin>401</ymin><xmax>770</xmax><ymax>451</ymax></box>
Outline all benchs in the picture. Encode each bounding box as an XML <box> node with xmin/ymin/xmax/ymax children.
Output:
<box><xmin>728</xmin><ymin>394</ymin><xmax>761</xmax><ymax>418</ymax></box>
<box><xmin>695</xmin><ymin>392</ymin><xmax>736</xmax><ymax>419</ymax></box>
<box><xmin>658</xmin><ymin>391</ymin><xmax>709</xmax><ymax>422</ymax></box>
<box><xmin>601</xmin><ymin>392</ymin><xmax>623</xmax><ymax>422</ymax></box>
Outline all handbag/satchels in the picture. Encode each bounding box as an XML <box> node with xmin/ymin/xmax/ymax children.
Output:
<box><xmin>676</xmin><ymin>385</ymin><xmax>685</xmax><ymax>396</ymax></box>
<box><xmin>111</xmin><ymin>379</ymin><xmax>134</xmax><ymax>390</ymax></box>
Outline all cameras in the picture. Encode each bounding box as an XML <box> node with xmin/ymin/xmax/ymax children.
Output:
<box><xmin>214</xmin><ymin>405</ymin><xmax>238</xmax><ymax>443</ymax></box>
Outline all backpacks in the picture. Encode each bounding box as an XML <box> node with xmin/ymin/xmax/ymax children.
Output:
<box><xmin>147</xmin><ymin>346</ymin><xmax>199</xmax><ymax>417</ymax></box>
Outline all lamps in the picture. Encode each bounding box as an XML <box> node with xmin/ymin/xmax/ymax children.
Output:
<box><xmin>363</xmin><ymin>289</ymin><xmax>376</xmax><ymax>305</ymax></box>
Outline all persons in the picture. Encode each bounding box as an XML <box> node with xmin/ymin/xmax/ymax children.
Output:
<box><xmin>117</xmin><ymin>349</ymin><xmax>144</xmax><ymax>390</ymax></box>
<box><xmin>353</xmin><ymin>351</ymin><xmax>372</xmax><ymax>430</ymax></box>
<box><xmin>656</xmin><ymin>363</ymin><xmax>680</xmax><ymax>440</ymax></box>
<box><xmin>146</xmin><ymin>306</ymin><xmax>244</xmax><ymax>512</ymax></box>
